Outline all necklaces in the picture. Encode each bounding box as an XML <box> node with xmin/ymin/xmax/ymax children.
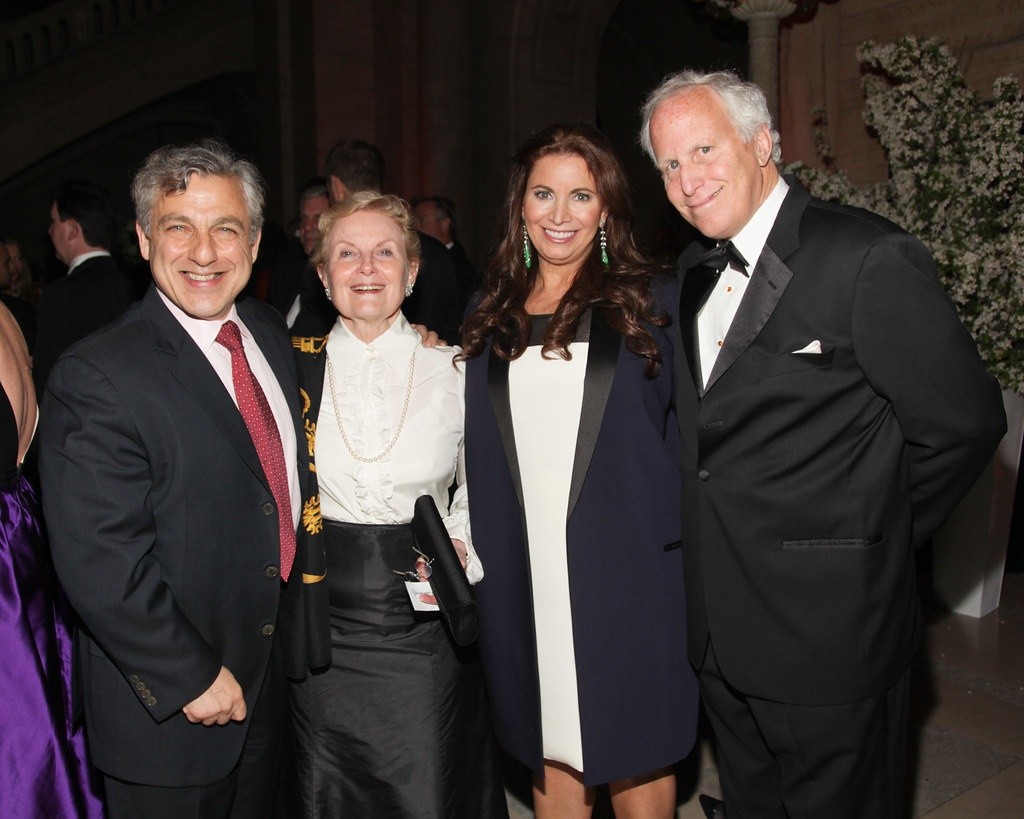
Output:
<box><xmin>325</xmin><ymin>342</ymin><xmax>415</xmax><ymax>464</ymax></box>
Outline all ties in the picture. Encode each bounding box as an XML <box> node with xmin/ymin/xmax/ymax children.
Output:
<box><xmin>214</xmin><ymin>319</ymin><xmax>298</xmax><ymax>583</ymax></box>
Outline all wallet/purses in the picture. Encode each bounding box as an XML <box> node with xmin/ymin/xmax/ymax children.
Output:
<box><xmin>408</xmin><ymin>495</ymin><xmax>482</xmax><ymax>666</ymax></box>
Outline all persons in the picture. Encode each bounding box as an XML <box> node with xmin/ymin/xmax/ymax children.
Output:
<box><xmin>0</xmin><ymin>175</ymin><xmax>147</xmax><ymax>406</ymax></box>
<box><xmin>0</xmin><ymin>302</ymin><xmax>107</xmax><ymax>818</ymax></box>
<box><xmin>290</xmin><ymin>190</ymin><xmax>485</xmax><ymax>819</ymax></box>
<box><xmin>38</xmin><ymin>143</ymin><xmax>447</xmax><ymax>819</ymax></box>
<box><xmin>640</xmin><ymin>70</ymin><xmax>1008</xmax><ymax>819</ymax></box>
<box><xmin>269</xmin><ymin>139</ymin><xmax>481</xmax><ymax>346</ymax></box>
<box><xmin>450</xmin><ymin>127</ymin><xmax>700</xmax><ymax>819</ymax></box>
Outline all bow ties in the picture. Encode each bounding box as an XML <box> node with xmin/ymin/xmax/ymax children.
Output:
<box><xmin>696</xmin><ymin>242</ymin><xmax>749</xmax><ymax>277</ymax></box>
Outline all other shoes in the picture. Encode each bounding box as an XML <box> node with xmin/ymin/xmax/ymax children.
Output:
<box><xmin>698</xmin><ymin>793</ymin><xmax>724</xmax><ymax>819</ymax></box>
